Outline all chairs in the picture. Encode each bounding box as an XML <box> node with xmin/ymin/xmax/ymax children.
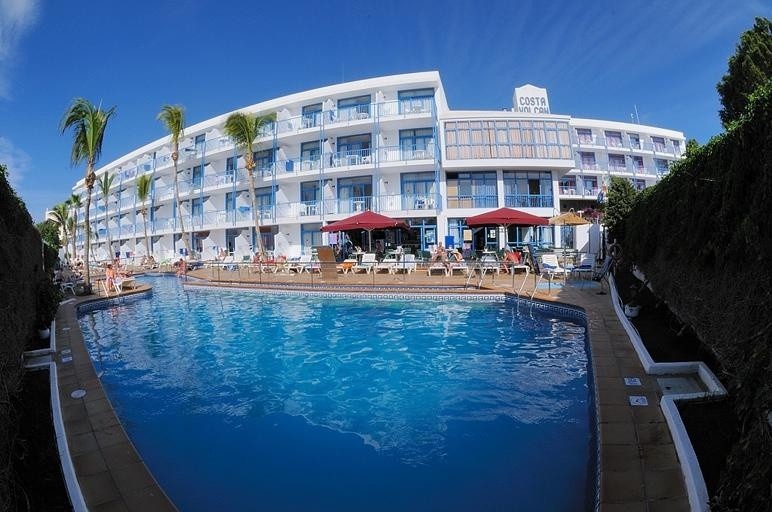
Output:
<box><xmin>204</xmin><ymin>245</ymin><xmax>416</xmax><ymax>275</ymax></box>
<box><xmin>428</xmin><ymin>252</ymin><xmax>615</xmax><ymax>293</ymax></box>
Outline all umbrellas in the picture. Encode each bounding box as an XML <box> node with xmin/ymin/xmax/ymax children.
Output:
<box><xmin>319</xmin><ymin>210</ymin><xmax>410</xmax><ymax>252</ymax></box>
<box><xmin>465</xmin><ymin>207</ymin><xmax>590</xmax><ymax>250</ymax></box>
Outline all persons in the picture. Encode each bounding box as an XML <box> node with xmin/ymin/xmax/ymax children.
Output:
<box><xmin>58</xmin><ymin>246</ymin><xmax>287</xmax><ymax>295</ymax></box>
<box><xmin>374</xmin><ymin>240</ymin><xmax>524</xmax><ymax>276</ymax></box>
